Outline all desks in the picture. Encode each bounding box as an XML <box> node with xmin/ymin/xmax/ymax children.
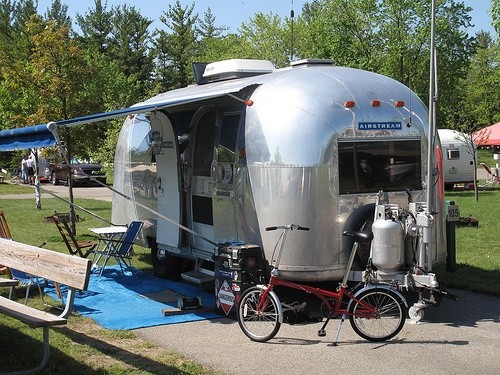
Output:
<box><xmin>87</xmin><ymin>226</ymin><xmax>132</xmax><ymax>277</ymax></box>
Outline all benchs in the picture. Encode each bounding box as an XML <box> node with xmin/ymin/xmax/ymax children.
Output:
<box><xmin>0</xmin><ymin>238</ymin><xmax>93</xmax><ymax>375</ymax></box>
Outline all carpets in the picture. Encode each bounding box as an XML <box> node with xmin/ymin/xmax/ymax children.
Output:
<box><xmin>5</xmin><ymin>264</ymin><xmax>226</xmax><ymax>332</ymax></box>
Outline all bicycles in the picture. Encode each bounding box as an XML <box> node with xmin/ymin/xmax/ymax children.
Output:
<box><xmin>236</xmin><ymin>225</ymin><xmax>410</xmax><ymax>347</ymax></box>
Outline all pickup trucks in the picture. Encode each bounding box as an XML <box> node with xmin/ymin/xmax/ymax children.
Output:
<box><xmin>49</xmin><ymin>158</ymin><xmax>107</xmax><ymax>188</ymax></box>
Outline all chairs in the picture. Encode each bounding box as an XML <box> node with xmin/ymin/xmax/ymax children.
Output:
<box><xmin>52</xmin><ymin>210</ymin><xmax>144</xmax><ymax>278</ymax></box>
<box><xmin>0</xmin><ymin>210</ymin><xmax>18</xmax><ymax>281</ymax></box>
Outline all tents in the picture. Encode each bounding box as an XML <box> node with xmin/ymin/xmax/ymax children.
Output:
<box><xmin>469</xmin><ymin>122</ymin><xmax>500</xmax><ymax>147</ymax></box>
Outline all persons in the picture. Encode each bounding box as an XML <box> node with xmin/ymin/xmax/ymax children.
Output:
<box><xmin>21</xmin><ymin>154</ymin><xmax>29</xmax><ymax>184</ymax></box>
<box><xmin>23</xmin><ymin>155</ymin><xmax>36</xmax><ymax>185</ymax></box>
<box><xmin>358</xmin><ymin>154</ymin><xmax>391</xmax><ymax>189</ymax></box>
